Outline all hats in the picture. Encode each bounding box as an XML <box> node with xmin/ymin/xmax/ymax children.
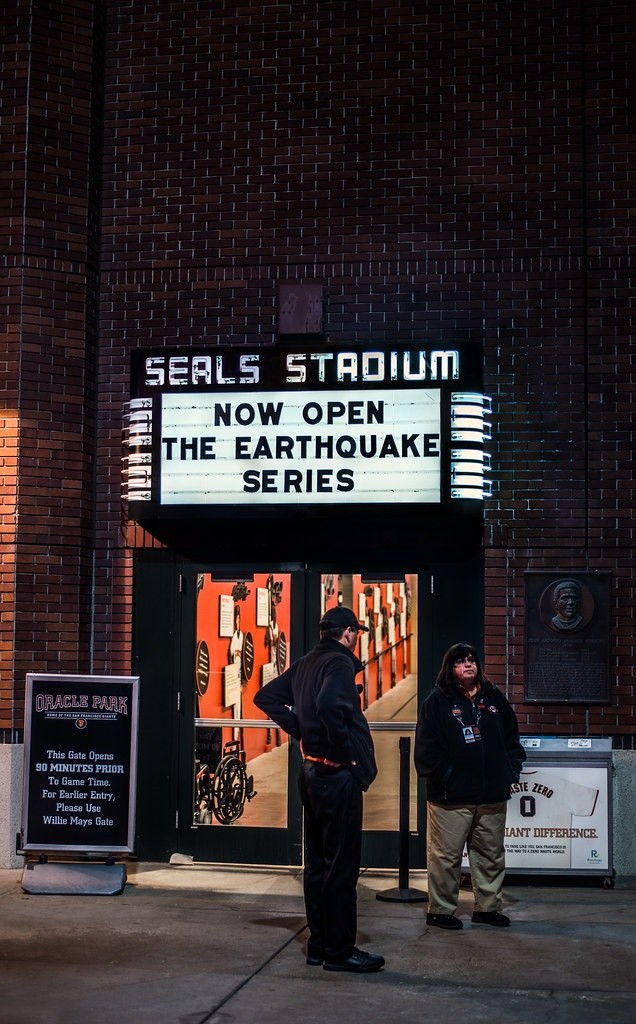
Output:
<box><xmin>320</xmin><ymin>606</ymin><xmax>369</xmax><ymax>631</ymax></box>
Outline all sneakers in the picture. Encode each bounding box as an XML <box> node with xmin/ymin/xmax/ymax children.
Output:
<box><xmin>425</xmin><ymin>913</ymin><xmax>463</xmax><ymax>928</ymax></box>
<box><xmin>306</xmin><ymin>948</ymin><xmax>386</xmax><ymax>972</ymax></box>
<box><xmin>471</xmin><ymin>911</ymin><xmax>511</xmax><ymax>927</ymax></box>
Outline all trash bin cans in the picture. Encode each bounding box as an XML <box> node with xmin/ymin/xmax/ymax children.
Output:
<box><xmin>462</xmin><ymin>736</ymin><xmax>616</xmax><ymax>889</ymax></box>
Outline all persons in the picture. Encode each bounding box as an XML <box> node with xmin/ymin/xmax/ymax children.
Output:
<box><xmin>552</xmin><ymin>582</ymin><xmax>582</xmax><ymax>629</ymax></box>
<box><xmin>252</xmin><ymin>607</ymin><xmax>386</xmax><ymax>972</ymax></box>
<box><xmin>414</xmin><ymin>644</ymin><xmax>528</xmax><ymax>930</ymax></box>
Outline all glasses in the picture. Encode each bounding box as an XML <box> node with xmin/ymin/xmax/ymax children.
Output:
<box><xmin>452</xmin><ymin>656</ymin><xmax>476</xmax><ymax>667</ymax></box>
